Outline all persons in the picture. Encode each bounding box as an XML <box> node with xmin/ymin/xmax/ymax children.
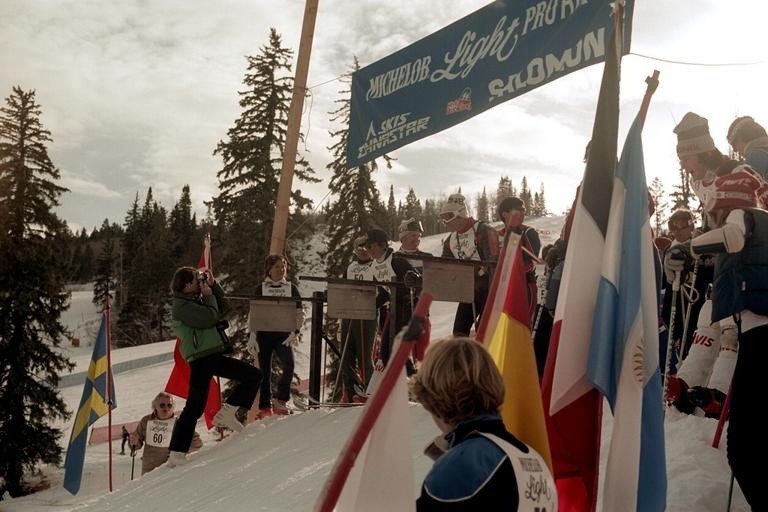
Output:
<box><xmin>406</xmin><ymin>335</ymin><xmax>559</xmax><ymax>512</ymax></box>
<box><xmin>127</xmin><ymin>389</ymin><xmax>203</xmax><ymax>481</ymax></box>
<box><xmin>164</xmin><ymin>263</ymin><xmax>264</xmax><ymax>466</ymax></box>
<box><xmin>324</xmin><ymin>193</ymin><xmax>568</xmax><ymax>406</ymax></box>
<box><xmin>246</xmin><ymin>255</ymin><xmax>303</xmax><ymax>417</ymax></box>
<box><xmin>649</xmin><ymin>112</ymin><xmax>768</xmax><ymax>512</ymax></box>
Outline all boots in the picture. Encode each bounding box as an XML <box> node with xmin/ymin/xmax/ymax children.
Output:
<box><xmin>167</xmin><ymin>451</ymin><xmax>187</xmax><ymax>467</ymax></box>
<box><xmin>213</xmin><ymin>404</ymin><xmax>243</xmax><ymax>431</ymax></box>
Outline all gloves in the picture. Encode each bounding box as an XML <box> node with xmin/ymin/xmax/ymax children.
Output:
<box><xmin>664</xmin><ymin>241</ymin><xmax>695</xmax><ymax>283</ymax></box>
<box><xmin>404</xmin><ymin>270</ymin><xmax>418</xmax><ymax>287</ymax></box>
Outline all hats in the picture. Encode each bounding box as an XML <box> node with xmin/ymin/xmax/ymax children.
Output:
<box><xmin>399</xmin><ymin>217</ymin><xmax>423</xmax><ymax>239</ymax></box>
<box><xmin>727</xmin><ymin>117</ymin><xmax>766</xmax><ymax>143</ymax></box>
<box><xmin>359</xmin><ymin>230</ymin><xmax>390</xmax><ymax>247</ymax></box>
<box><xmin>440</xmin><ymin>194</ymin><xmax>469</xmax><ymax>219</ymax></box>
<box><xmin>674</xmin><ymin>112</ymin><xmax>714</xmax><ymax>156</ymax></box>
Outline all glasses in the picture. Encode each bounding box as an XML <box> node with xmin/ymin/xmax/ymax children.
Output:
<box><xmin>158</xmin><ymin>402</ymin><xmax>171</xmax><ymax>409</ymax></box>
<box><xmin>439</xmin><ymin>206</ymin><xmax>466</xmax><ymax>224</ymax></box>
<box><xmin>400</xmin><ymin>221</ymin><xmax>424</xmax><ymax>232</ymax></box>
<box><xmin>669</xmin><ymin>219</ymin><xmax>695</xmax><ymax>230</ymax></box>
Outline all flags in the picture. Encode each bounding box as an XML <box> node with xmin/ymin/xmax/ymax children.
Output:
<box><xmin>352</xmin><ymin>321</ymin><xmax>440</xmax><ymax>512</ymax></box>
<box><xmin>164</xmin><ymin>232</ymin><xmax>223</xmax><ymax>431</ymax></box>
<box><xmin>62</xmin><ymin>309</ymin><xmax>118</xmax><ymax>496</ymax></box>
<box><xmin>586</xmin><ymin>118</ymin><xmax>668</xmax><ymax>511</ymax></box>
<box><xmin>424</xmin><ymin>227</ymin><xmax>554</xmax><ymax>489</ymax></box>
<box><xmin>539</xmin><ymin>4</ymin><xmax>623</xmax><ymax>512</ymax></box>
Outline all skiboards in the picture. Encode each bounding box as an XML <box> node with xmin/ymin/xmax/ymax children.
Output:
<box><xmin>273</xmin><ymin>394</ymin><xmax>364</xmax><ymax>413</ymax></box>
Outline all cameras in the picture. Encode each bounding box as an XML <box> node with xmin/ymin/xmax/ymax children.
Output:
<box><xmin>197</xmin><ymin>272</ymin><xmax>208</xmax><ymax>281</ymax></box>
